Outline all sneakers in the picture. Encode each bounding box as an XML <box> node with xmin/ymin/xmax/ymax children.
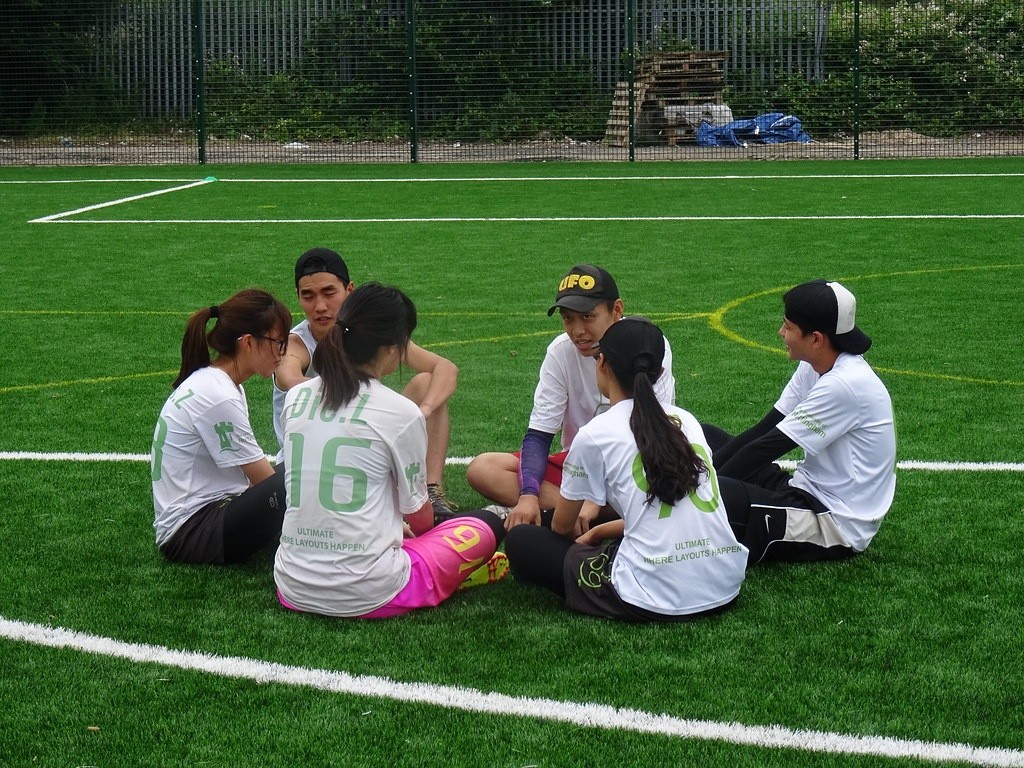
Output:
<box><xmin>425</xmin><ymin>483</ymin><xmax>460</xmax><ymax>521</ymax></box>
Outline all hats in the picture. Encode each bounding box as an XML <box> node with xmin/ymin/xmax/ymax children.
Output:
<box><xmin>784</xmin><ymin>279</ymin><xmax>872</xmax><ymax>356</ymax></box>
<box><xmin>599</xmin><ymin>317</ymin><xmax>664</xmax><ymax>383</ymax></box>
<box><xmin>295</xmin><ymin>248</ymin><xmax>350</xmax><ymax>286</ymax></box>
<box><xmin>547</xmin><ymin>264</ymin><xmax>619</xmax><ymax>316</ymax></box>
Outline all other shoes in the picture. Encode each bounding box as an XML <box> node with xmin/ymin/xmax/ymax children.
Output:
<box><xmin>456</xmin><ymin>552</ymin><xmax>511</xmax><ymax>590</ymax></box>
<box><xmin>482</xmin><ymin>504</ymin><xmax>516</xmax><ymax>521</ymax></box>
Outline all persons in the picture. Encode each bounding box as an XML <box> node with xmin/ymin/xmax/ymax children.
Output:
<box><xmin>503</xmin><ymin>316</ymin><xmax>750</xmax><ymax>622</ymax></box>
<box><xmin>466</xmin><ymin>265</ymin><xmax>677</xmax><ymax>534</ymax></box>
<box><xmin>700</xmin><ymin>279</ymin><xmax>897</xmax><ymax>569</ymax></box>
<box><xmin>271</xmin><ymin>246</ymin><xmax>459</xmax><ymax>528</ymax></box>
<box><xmin>151</xmin><ymin>289</ymin><xmax>288</xmax><ymax>566</ymax></box>
<box><xmin>272</xmin><ymin>282</ymin><xmax>508</xmax><ymax>618</ymax></box>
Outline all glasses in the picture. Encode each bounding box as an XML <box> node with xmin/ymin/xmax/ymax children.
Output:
<box><xmin>238</xmin><ymin>332</ymin><xmax>285</xmax><ymax>356</ymax></box>
